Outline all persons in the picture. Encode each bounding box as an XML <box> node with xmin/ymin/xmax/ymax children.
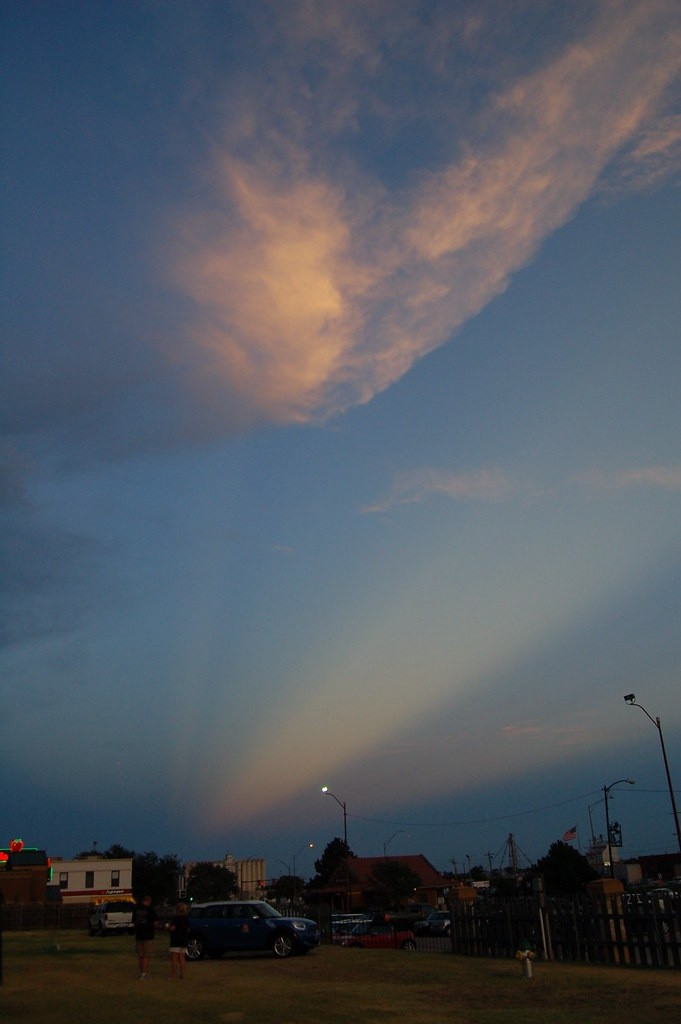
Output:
<box><xmin>227</xmin><ymin>891</ymin><xmax>238</xmax><ymax>901</ymax></box>
<box><xmin>166</xmin><ymin>903</ymin><xmax>192</xmax><ymax>980</ymax></box>
<box><xmin>132</xmin><ymin>897</ymin><xmax>161</xmax><ymax>981</ymax></box>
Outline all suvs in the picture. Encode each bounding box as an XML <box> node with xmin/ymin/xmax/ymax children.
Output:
<box><xmin>89</xmin><ymin>901</ymin><xmax>134</xmax><ymax>937</ymax></box>
<box><xmin>186</xmin><ymin>901</ymin><xmax>322</xmax><ymax>957</ymax></box>
<box><xmin>332</xmin><ymin>904</ymin><xmax>450</xmax><ymax>951</ymax></box>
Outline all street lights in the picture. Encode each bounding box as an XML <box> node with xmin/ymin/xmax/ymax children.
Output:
<box><xmin>603</xmin><ymin>779</ymin><xmax>635</xmax><ymax>879</ymax></box>
<box><xmin>321</xmin><ymin>787</ymin><xmax>346</xmax><ymax>913</ymax></box>
<box><xmin>293</xmin><ymin>843</ymin><xmax>314</xmax><ymax>887</ymax></box>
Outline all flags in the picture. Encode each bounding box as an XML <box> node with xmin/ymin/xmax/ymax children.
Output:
<box><xmin>562</xmin><ymin>824</ymin><xmax>577</xmax><ymax>841</ymax></box>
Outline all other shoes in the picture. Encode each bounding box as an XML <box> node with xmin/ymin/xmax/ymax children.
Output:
<box><xmin>168</xmin><ymin>978</ymin><xmax>176</xmax><ymax>982</ymax></box>
<box><xmin>139</xmin><ymin>974</ymin><xmax>154</xmax><ymax>980</ymax></box>
